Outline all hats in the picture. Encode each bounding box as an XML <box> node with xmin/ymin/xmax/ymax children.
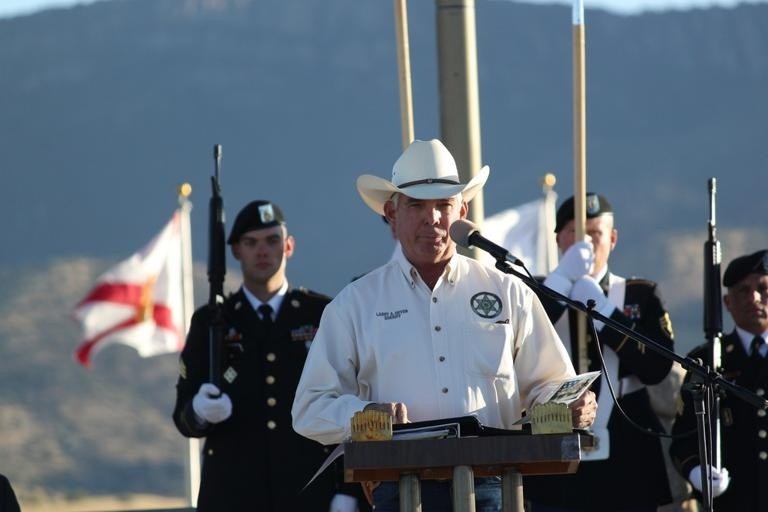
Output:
<box><xmin>723</xmin><ymin>250</ymin><xmax>768</xmax><ymax>286</ymax></box>
<box><xmin>227</xmin><ymin>200</ymin><xmax>285</xmax><ymax>244</ymax></box>
<box><xmin>355</xmin><ymin>137</ymin><xmax>490</xmax><ymax>217</ymax></box>
<box><xmin>554</xmin><ymin>192</ymin><xmax>612</xmax><ymax>232</ymax></box>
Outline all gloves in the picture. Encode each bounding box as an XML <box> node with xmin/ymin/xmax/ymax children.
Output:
<box><xmin>569</xmin><ymin>274</ymin><xmax>606</xmax><ymax>317</ymax></box>
<box><xmin>192</xmin><ymin>383</ymin><xmax>233</xmax><ymax>425</ymax></box>
<box><xmin>555</xmin><ymin>239</ymin><xmax>594</xmax><ymax>281</ymax></box>
<box><xmin>688</xmin><ymin>463</ymin><xmax>731</xmax><ymax>497</ymax></box>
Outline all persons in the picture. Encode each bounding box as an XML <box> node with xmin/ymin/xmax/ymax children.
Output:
<box><xmin>168</xmin><ymin>194</ymin><xmax>363</xmax><ymax>512</ymax></box>
<box><xmin>0</xmin><ymin>473</ymin><xmax>21</xmax><ymax>511</ymax></box>
<box><xmin>667</xmin><ymin>247</ymin><xmax>768</xmax><ymax>512</ymax></box>
<box><xmin>291</xmin><ymin>136</ymin><xmax>599</xmax><ymax>512</ymax></box>
<box><xmin>529</xmin><ymin>190</ymin><xmax>674</xmax><ymax>512</ymax></box>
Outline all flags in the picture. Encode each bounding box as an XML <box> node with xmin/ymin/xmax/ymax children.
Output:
<box><xmin>72</xmin><ymin>200</ymin><xmax>197</xmax><ymax>358</ymax></box>
<box><xmin>478</xmin><ymin>189</ymin><xmax>575</xmax><ymax>360</ymax></box>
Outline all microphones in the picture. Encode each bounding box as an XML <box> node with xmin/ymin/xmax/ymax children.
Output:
<box><xmin>451</xmin><ymin>219</ymin><xmax>525</xmax><ymax>269</ymax></box>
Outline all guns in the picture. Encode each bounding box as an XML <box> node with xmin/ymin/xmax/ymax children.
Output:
<box><xmin>206</xmin><ymin>143</ymin><xmax>235</xmax><ymax>398</ymax></box>
<box><xmin>703</xmin><ymin>176</ymin><xmax>723</xmax><ymax>469</ymax></box>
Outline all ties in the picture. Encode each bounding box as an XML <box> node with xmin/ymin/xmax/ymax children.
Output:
<box><xmin>259</xmin><ymin>305</ymin><xmax>273</xmax><ymax>323</ymax></box>
<box><xmin>750</xmin><ymin>336</ymin><xmax>765</xmax><ymax>361</ymax></box>
